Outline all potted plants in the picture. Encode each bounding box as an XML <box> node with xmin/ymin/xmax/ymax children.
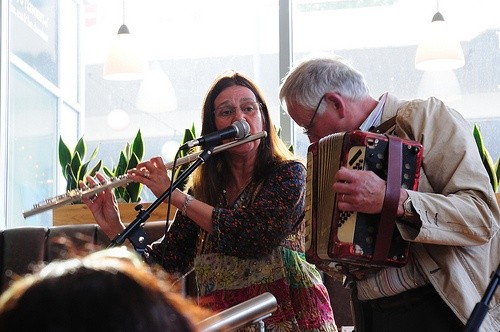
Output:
<box><xmin>52</xmin><ymin>123</ymin><xmax>200</xmax><ymax>227</ymax></box>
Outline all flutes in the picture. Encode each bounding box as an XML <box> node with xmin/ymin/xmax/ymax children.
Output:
<box><xmin>23</xmin><ymin>131</ymin><xmax>266</xmax><ymax>218</ymax></box>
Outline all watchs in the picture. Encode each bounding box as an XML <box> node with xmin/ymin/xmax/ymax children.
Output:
<box><xmin>181</xmin><ymin>192</ymin><xmax>195</xmax><ymax>218</ymax></box>
<box><xmin>397</xmin><ymin>196</ymin><xmax>417</xmax><ymax>222</ymax></box>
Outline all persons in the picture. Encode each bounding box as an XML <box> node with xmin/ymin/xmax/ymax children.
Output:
<box><xmin>0</xmin><ymin>245</ymin><xmax>204</xmax><ymax>332</ymax></box>
<box><xmin>78</xmin><ymin>71</ymin><xmax>340</xmax><ymax>332</ymax></box>
<box><xmin>278</xmin><ymin>57</ymin><xmax>500</xmax><ymax>332</ymax></box>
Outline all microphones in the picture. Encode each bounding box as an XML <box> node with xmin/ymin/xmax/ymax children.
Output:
<box><xmin>179</xmin><ymin>120</ymin><xmax>250</xmax><ymax>150</ymax></box>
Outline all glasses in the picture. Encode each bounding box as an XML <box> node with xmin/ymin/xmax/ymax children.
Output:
<box><xmin>212</xmin><ymin>102</ymin><xmax>263</xmax><ymax>119</ymax></box>
<box><xmin>302</xmin><ymin>92</ymin><xmax>341</xmax><ymax>137</ymax></box>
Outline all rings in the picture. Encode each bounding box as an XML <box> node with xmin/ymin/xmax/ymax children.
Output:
<box><xmin>341</xmin><ymin>194</ymin><xmax>345</xmax><ymax>202</ymax></box>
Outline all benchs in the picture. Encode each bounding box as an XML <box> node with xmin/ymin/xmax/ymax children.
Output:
<box><xmin>0</xmin><ymin>220</ymin><xmax>171</xmax><ymax>300</ymax></box>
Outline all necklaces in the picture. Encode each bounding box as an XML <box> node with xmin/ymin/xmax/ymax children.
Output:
<box><xmin>225</xmin><ymin>174</ymin><xmax>253</xmax><ymax>203</ymax></box>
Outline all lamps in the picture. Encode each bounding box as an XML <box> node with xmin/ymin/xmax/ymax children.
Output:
<box><xmin>414</xmin><ymin>0</ymin><xmax>466</xmax><ymax>71</ymax></box>
